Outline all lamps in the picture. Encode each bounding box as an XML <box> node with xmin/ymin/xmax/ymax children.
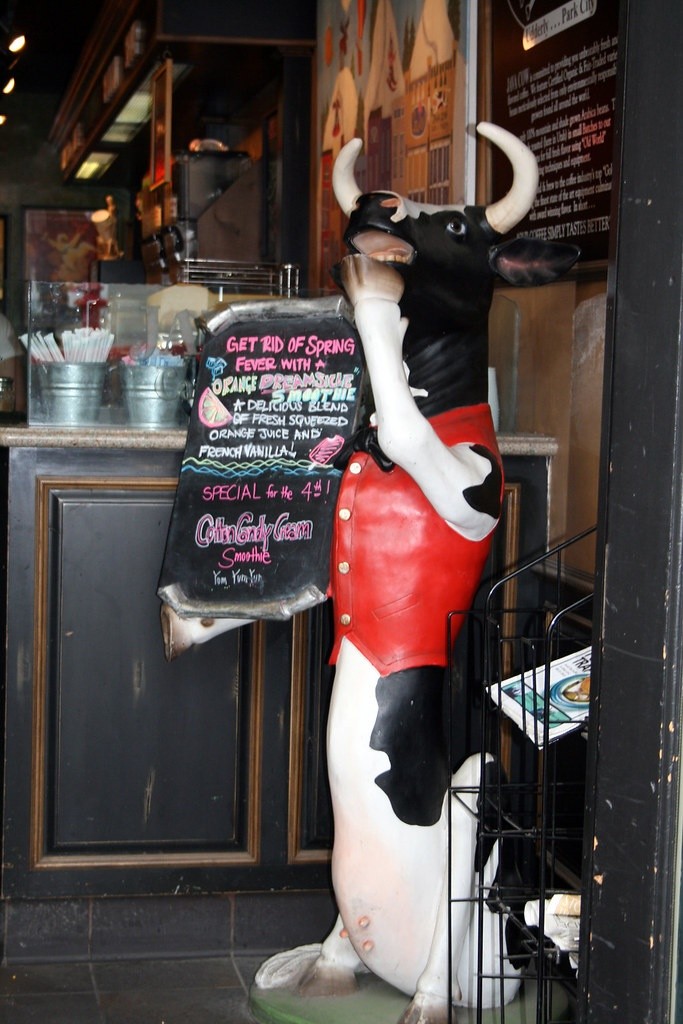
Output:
<box><xmin>0</xmin><ymin>68</ymin><xmax>16</xmax><ymax>94</ymax></box>
<box><xmin>0</xmin><ymin>42</ymin><xmax>21</xmax><ymax>70</ymax></box>
<box><xmin>0</xmin><ymin>16</ymin><xmax>27</xmax><ymax>53</ymax></box>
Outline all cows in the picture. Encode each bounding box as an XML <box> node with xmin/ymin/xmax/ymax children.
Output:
<box><xmin>156</xmin><ymin>119</ymin><xmax>540</xmax><ymax>1024</ymax></box>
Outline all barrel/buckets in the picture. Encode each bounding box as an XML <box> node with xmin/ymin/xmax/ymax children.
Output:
<box><xmin>109</xmin><ymin>364</ymin><xmax>193</xmax><ymax>429</ymax></box>
<box><xmin>35</xmin><ymin>361</ymin><xmax>118</xmax><ymax>424</ymax></box>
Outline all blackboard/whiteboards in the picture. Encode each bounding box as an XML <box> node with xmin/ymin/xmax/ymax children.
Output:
<box><xmin>156</xmin><ymin>316</ymin><xmax>368</xmax><ymax>619</ymax></box>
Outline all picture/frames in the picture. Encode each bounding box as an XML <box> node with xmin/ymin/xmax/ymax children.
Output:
<box><xmin>0</xmin><ymin>213</ymin><xmax>11</xmax><ymax>281</ymax></box>
<box><xmin>21</xmin><ymin>204</ymin><xmax>101</xmax><ymax>331</ymax></box>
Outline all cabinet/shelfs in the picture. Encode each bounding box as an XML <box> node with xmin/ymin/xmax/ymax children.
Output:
<box><xmin>446</xmin><ymin>524</ymin><xmax>601</xmax><ymax>1024</ymax></box>
<box><xmin>48</xmin><ymin>0</ymin><xmax>317</xmax><ymax>296</ymax></box>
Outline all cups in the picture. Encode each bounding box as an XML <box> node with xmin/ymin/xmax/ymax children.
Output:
<box><xmin>91</xmin><ymin>209</ymin><xmax>113</xmax><ymax>242</ymax></box>
